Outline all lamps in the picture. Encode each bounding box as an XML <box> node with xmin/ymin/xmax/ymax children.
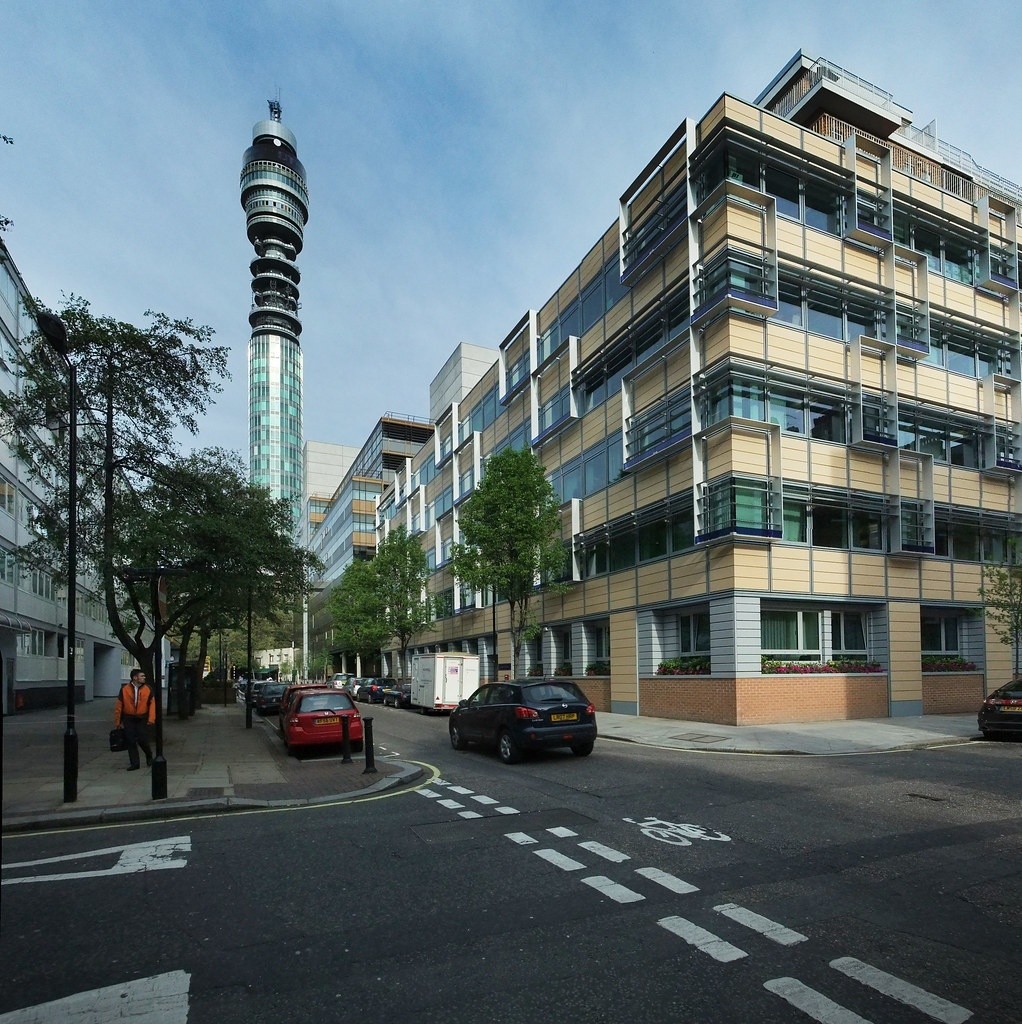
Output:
<box><xmin>543</xmin><ymin>625</ymin><xmax>549</xmax><ymax>632</ymax></box>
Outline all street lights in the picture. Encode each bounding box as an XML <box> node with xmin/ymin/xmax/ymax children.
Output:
<box><xmin>38</xmin><ymin>311</ymin><xmax>81</xmax><ymax>802</ymax></box>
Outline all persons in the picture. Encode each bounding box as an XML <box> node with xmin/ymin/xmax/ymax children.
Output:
<box><xmin>114</xmin><ymin>670</ymin><xmax>156</xmax><ymax>771</ymax></box>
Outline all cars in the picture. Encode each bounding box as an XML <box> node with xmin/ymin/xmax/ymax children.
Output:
<box><xmin>256</xmin><ymin>682</ymin><xmax>291</xmax><ymax>717</ymax></box>
<box><xmin>280</xmin><ymin>683</ymin><xmax>364</xmax><ymax>758</ymax></box>
<box><xmin>978</xmin><ymin>677</ymin><xmax>1022</xmax><ymax>742</ymax></box>
<box><xmin>326</xmin><ymin>672</ymin><xmax>411</xmax><ymax>709</ymax></box>
<box><xmin>238</xmin><ymin>678</ymin><xmax>260</xmax><ymax>703</ymax></box>
<box><xmin>449</xmin><ymin>678</ymin><xmax>598</xmax><ymax>765</ymax></box>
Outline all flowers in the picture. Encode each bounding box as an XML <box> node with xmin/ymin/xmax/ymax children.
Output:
<box><xmin>761</xmin><ymin>656</ymin><xmax>884</xmax><ymax>675</ymax></box>
<box><xmin>554</xmin><ymin>661</ymin><xmax>572</xmax><ymax>676</ymax></box>
<box><xmin>527</xmin><ymin>664</ymin><xmax>543</xmax><ymax>677</ymax></box>
<box><xmin>655</xmin><ymin>657</ymin><xmax>711</xmax><ymax>675</ymax></box>
<box><xmin>585</xmin><ymin>660</ymin><xmax>611</xmax><ymax>676</ymax></box>
<box><xmin>922</xmin><ymin>655</ymin><xmax>977</xmax><ymax>672</ymax></box>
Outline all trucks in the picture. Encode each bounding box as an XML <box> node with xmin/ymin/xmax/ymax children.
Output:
<box><xmin>410</xmin><ymin>651</ymin><xmax>481</xmax><ymax>714</ymax></box>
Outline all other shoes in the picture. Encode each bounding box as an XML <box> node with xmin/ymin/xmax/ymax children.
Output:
<box><xmin>127</xmin><ymin>763</ymin><xmax>140</xmax><ymax>771</ymax></box>
<box><xmin>146</xmin><ymin>751</ymin><xmax>154</xmax><ymax>766</ymax></box>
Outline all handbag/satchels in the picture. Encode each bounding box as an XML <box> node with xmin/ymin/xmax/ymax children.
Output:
<box><xmin>110</xmin><ymin>726</ymin><xmax>128</xmax><ymax>752</ymax></box>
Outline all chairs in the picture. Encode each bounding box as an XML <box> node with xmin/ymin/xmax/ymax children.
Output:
<box><xmin>324</xmin><ymin>696</ymin><xmax>342</xmax><ymax>709</ymax></box>
<box><xmin>532</xmin><ymin>688</ymin><xmax>541</xmax><ymax>698</ymax></box>
<box><xmin>301</xmin><ymin>699</ymin><xmax>314</xmax><ymax>711</ymax></box>
<box><xmin>492</xmin><ymin>690</ymin><xmax>510</xmax><ymax>703</ymax></box>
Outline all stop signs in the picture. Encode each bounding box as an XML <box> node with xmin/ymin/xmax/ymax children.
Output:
<box><xmin>158</xmin><ymin>574</ymin><xmax>167</xmax><ymax>622</ymax></box>
